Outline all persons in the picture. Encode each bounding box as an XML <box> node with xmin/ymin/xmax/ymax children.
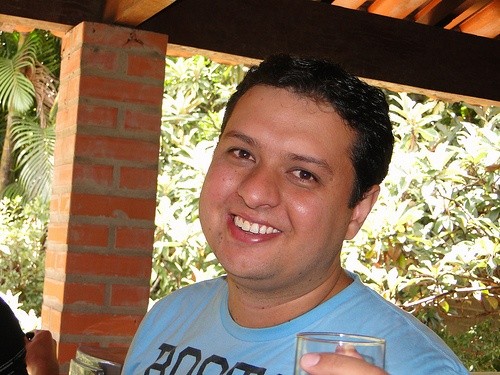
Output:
<box><xmin>24</xmin><ymin>328</ymin><xmax>60</xmax><ymax>375</ymax></box>
<box><xmin>116</xmin><ymin>54</ymin><xmax>467</xmax><ymax>375</ymax></box>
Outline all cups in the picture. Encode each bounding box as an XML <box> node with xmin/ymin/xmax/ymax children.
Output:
<box><xmin>294</xmin><ymin>329</ymin><xmax>387</xmax><ymax>375</ymax></box>
